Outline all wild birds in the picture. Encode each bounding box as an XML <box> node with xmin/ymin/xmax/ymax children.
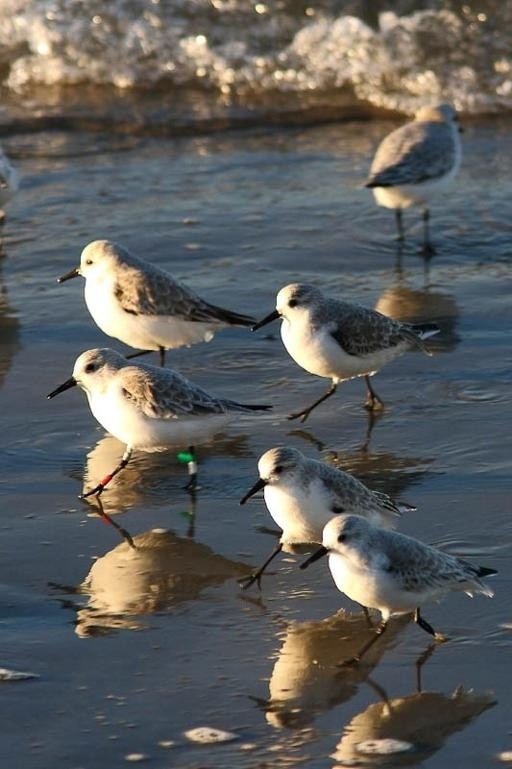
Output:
<box><xmin>43</xmin><ymin>347</ymin><xmax>280</xmax><ymax>505</ymax></box>
<box><xmin>245</xmin><ymin>603</ymin><xmax>422</xmax><ymax>730</ymax></box>
<box><xmin>250</xmin><ymin>279</ymin><xmax>437</xmax><ymax>419</ymax></box>
<box><xmin>44</xmin><ymin>505</ymin><xmax>255</xmax><ymax>638</ymax></box>
<box><xmin>51</xmin><ymin>237</ymin><xmax>260</xmax><ymax>372</ymax></box>
<box><xmin>61</xmin><ymin>436</ymin><xmax>251</xmax><ymax>518</ymax></box>
<box><xmin>326</xmin><ymin>679</ymin><xmax>500</xmax><ymax>767</ymax></box>
<box><xmin>233</xmin><ymin>449</ymin><xmax>425</xmax><ymax>595</ymax></box>
<box><xmin>353</xmin><ymin>95</ymin><xmax>464</xmax><ymax>254</ymax></box>
<box><xmin>298</xmin><ymin>510</ymin><xmax>501</xmax><ymax>668</ymax></box>
<box><xmin>372</xmin><ymin>255</ymin><xmax>460</xmax><ymax>359</ymax></box>
